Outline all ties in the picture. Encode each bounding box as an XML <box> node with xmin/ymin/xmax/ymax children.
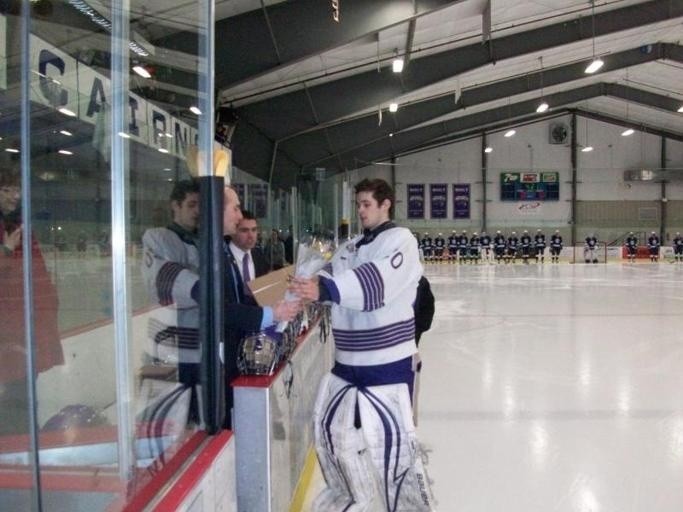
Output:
<box><xmin>242</xmin><ymin>253</ymin><xmax>250</xmax><ymax>296</ymax></box>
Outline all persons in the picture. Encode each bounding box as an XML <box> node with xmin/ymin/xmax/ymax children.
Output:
<box><xmin>420</xmin><ymin>228</ymin><xmax>564</xmax><ymax>266</ymax></box>
<box><xmin>220</xmin><ymin>187</ymin><xmax>302</xmax><ymax>434</ymax></box>
<box><xmin>264</xmin><ymin>227</ymin><xmax>287</xmax><ymax>270</ymax></box>
<box><xmin>583</xmin><ymin>232</ymin><xmax>599</xmax><ymax>264</ymax></box>
<box><xmin>226</xmin><ymin>209</ymin><xmax>270</xmax><ymax>286</ymax></box>
<box><xmin>284</xmin><ymin>178</ymin><xmax>443</xmax><ymax>512</ymax></box>
<box><xmin>647</xmin><ymin>230</ymin><xmax>661</xmax><ymax>263</ymax></box>
<box><xmin>134</xmin><ymin>179</ymin><xmax>207</xmax><ymax>477</ymax></box>
<box><xmin>624</xmin><ymin>230</ymin><xmax>640</xmax><ymax>264</ymax></box>
<box><xmin>0</xmin><ymin>168</ymin><xmax>69</xmax><ymax>435</ymax></box>
<box><xmin>673</xmin><ymin>231</ymin><xmax>683</xmax><ymax>264</ymax></box>
